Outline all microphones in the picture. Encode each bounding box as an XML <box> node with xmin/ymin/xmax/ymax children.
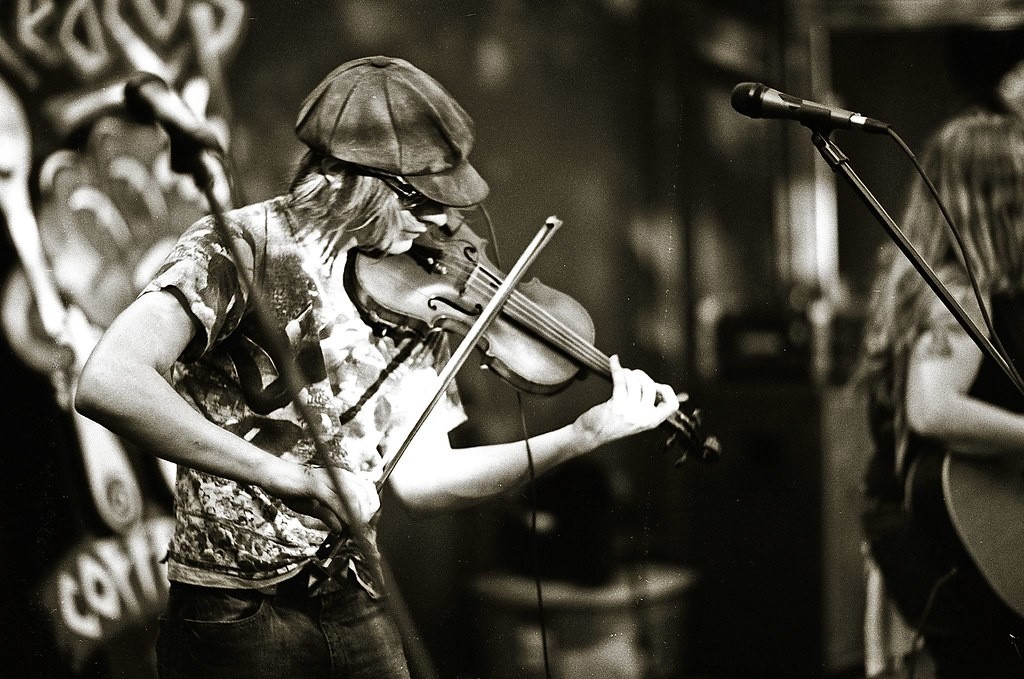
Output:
<box><xmin>730</xmin><ymin>83</ymin><xmax>891</xmax><ymax>136</ymax></box>
<box><xmin>124</xmin><ymin>71</ymin><xmax>224</xmax><ymax>154</ymax></box>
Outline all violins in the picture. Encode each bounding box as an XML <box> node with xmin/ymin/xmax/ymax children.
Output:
<box><xmin>341</xmin><ymin>207</ymin><xmax>722</xmax><ymax>472</ymax></box>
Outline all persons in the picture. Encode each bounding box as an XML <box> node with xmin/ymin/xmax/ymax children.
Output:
<box><xmin>75</xmin><ymin>55</ymin><xmax>680</xmax><ymax>679</ymax></box>
<box><xmin>860</xmin><ymin>112</ymin><xmax>1024</xmax><ymax>679</ymax></box>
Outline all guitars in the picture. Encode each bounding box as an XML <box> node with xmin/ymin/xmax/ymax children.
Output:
<box><xmin>858</xmin><ymin>424</ymin><xmax>1024</xmax><ymax>679</ymax></box>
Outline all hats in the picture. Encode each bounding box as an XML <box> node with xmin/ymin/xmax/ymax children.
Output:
<box><xmin>294</xmin><ymin>55</ymin><xmax>489</xmax><ymax>207</ymax></box>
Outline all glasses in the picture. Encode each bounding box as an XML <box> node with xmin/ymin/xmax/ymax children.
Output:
<box><xmin>373</xmin><ymin>175</ymin><xmax>428</xmax><ymax>208</ymax></box>
